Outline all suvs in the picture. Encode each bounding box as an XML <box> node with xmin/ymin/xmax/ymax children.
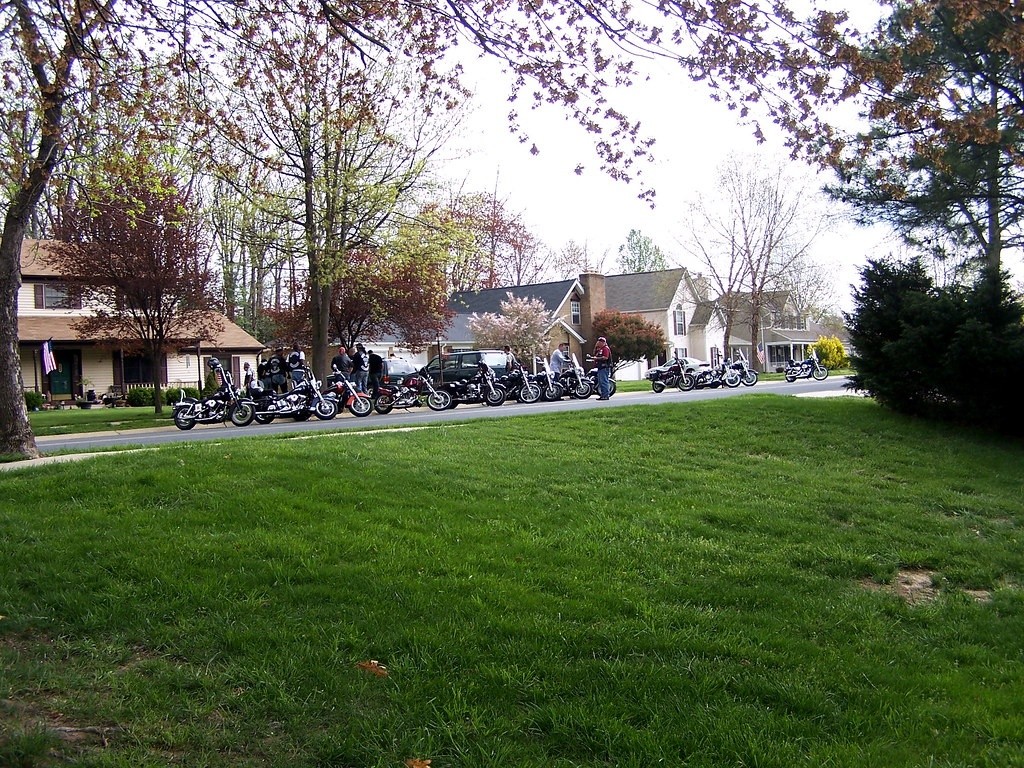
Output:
<box><xmin>326</xmin><ymin>357</ymin><xmax>418</xmax><ymax>395</ymax></box>
<box><xmin>401</xmin><ymin>349</ymin><xmax>517</xmax><ymax>395</ymax></box>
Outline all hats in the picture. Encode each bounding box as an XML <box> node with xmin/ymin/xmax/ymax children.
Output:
<box><xmin>598</xmin><ymin>336</ymin><xmax>606</xmax><ymax>342</ymax></box>
<box><xmin>261</xmin><ymin>359</ymin><xmax>267</xmax><ymax>362</ymax></box>
<box><xmin>244</xmin><ymin>362</ymin><xmax>250</xmax><ymax>368</ymax></box>
<box><xmin>276</xmin><ymin>347</ymin><xmax>283</xmax><ymax>351</ymax></box>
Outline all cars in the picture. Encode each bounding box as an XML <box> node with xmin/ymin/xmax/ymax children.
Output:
<box><xmin>644</xmin><ymin>356</ymin><xmax>712</xmax><ymax>380</ymax></box>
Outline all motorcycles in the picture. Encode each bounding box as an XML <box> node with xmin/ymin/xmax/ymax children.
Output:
<box><xmin>539</xmin><ymin>352</ymin><xmax>596</xmax><ymax>401</ymax></box>
<box><xmin>693</xmin><ymin>353</ymin><xmax>742</xmax><ymax>390</ymax></box>
<box><xmin>374</xmin><ymin>366</ymin><xmax>452</xmax><ymax>415</ymax></box>
<box><xmin>709</xmin><ymin>348</ymin><xmax>759</xmax><ymax>388</ymax></box>
<box><xmin>171</xmin><ymin>364</ymin><xmax>256</xmax><ymax>430</ymax></box>
<box><xmin>247</xmin><ymin>359</ymin><xmax>340</xmax><ymax>424</ymax></box>
<box><xmin>435</xmin><ymin>359</ymin><xmax>507</xmax><ymax>409</ymax></box>
<box><xmin>573</xmin><ymin>353</ymin><xmax>616</xmax><ymax>398</ymax></box>
<box><xmin>784</xmin><ymin>348</ymin><xmax>829</xmax><ymax>383</ymax></box>
<box><xmin>648</xmin><ymin>349</ymin><xmax>696</xmax><ymax>393</ymax></box>
<box><xmin>485</xmin><ymin>354</ymin><xmax>542</xmax><ymax>404</ymax></box>
<box><xmin>522</xmin><ymin>357</ymin><xmax>565</xmax><ymax>402</ymax></box>
<box><xmin>293</xmin><ymin>362</ymin><xmax>373</xmax><ymax>421</ymax></box>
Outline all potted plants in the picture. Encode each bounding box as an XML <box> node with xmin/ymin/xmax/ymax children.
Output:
<box><xmin>79</xmin><ymin>377</ymin><xmax>93</xmax><ymax>409</ymax></box>
<box><xmin>115</xmin><ymin>400</ymin><xmax>126</xmax><ymax>406</ymax></box>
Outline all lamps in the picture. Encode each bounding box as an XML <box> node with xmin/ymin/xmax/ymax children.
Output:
<box><xmin>61</xmin><ymin>400</ymin><xmax>65</xmax><ymax>406</ymax></box>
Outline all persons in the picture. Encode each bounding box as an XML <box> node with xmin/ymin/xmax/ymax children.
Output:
<box><xmin>503</xmin><ymin>346</ymin><xmax>516</xmax><ymax>373</ymax></box>
<box><xmin>551</xmin><ymin>343</ymin><xmax>577</xmax><ymax>401</ymax></box>
<box><xmin>332</xmin><ymin>347</ymin><xmax>385</xmax><ymax>400</ymax></box>
<box><xmin>210</xmin><ymin>341</ymin><xmax>307</xmax><ymax>393</ymax></box>
<box><xmin>587</xmin><ymin>338</ymin><xmax>613</xmax><ymax>400</ymax></box>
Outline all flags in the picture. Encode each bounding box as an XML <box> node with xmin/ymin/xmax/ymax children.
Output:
<box><xmin>756</xmin><ymin>342</ymin><xmax>765</xmax><ymax>363</ymax></box>
<box><xmin>43</xmin><ymin>339</ymin><xmax>57</xmax><ymax>374</ymax></box>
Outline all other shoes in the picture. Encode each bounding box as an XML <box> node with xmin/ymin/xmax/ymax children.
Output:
<box><xmin>596</xmin><ymin>396</ymin><xmax>609</xmax><ymax>400</ymax></box>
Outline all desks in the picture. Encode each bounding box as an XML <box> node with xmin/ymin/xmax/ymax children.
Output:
<box><xmin>85</xmin><ymin>391</ymin><xmax>97</xmax><ymax>401</ymax></box>
<box><xmin>111</xmin><ymin>384</ymin><xmax>122</xmax><ymax>398</ymax></box>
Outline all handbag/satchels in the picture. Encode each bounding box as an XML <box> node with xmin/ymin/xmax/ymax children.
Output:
<box><xmin>598</xmin><ymin>353</ymin><xmax>613</xmax><ymax>370</ymax></box>
